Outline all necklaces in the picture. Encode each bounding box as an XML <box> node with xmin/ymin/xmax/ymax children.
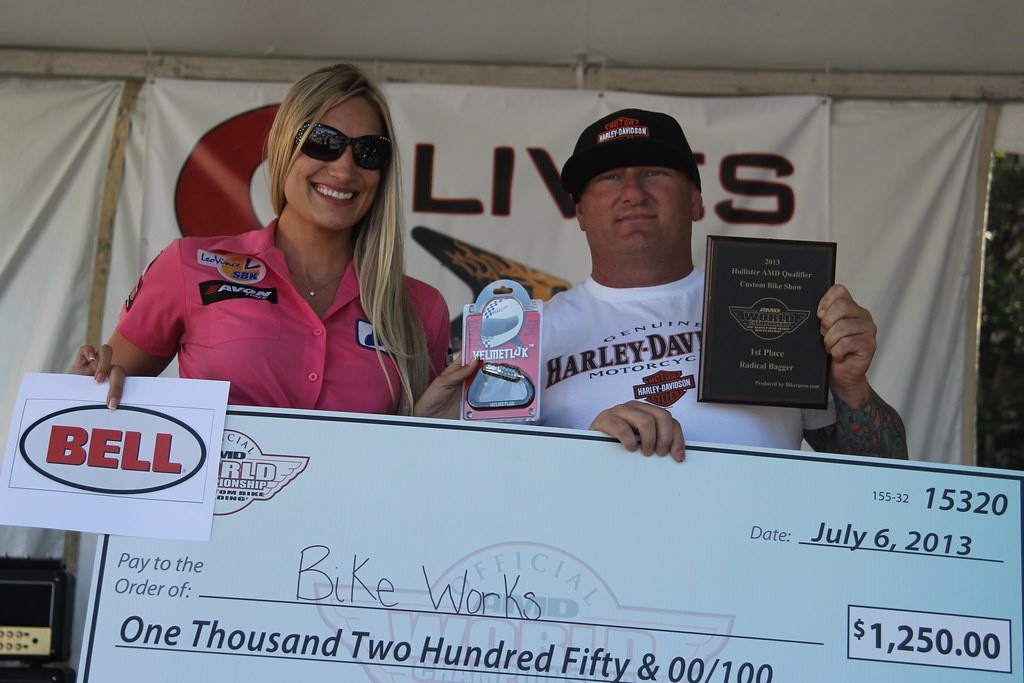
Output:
<box><xmin>290</xmin><ymin>272</ymin><xmax>343</xmax><ymax>295</ymax></box>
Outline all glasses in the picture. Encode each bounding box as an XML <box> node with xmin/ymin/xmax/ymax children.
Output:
<box><xmin>294</xmin><ymin>122</ymin><xmax>394</xmax><ymax>170</ymax></box>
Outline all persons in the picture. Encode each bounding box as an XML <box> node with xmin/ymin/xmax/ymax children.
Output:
<box><xmin>67</xmin><ymin>65</ymin><xmax>477</xmax><ymax>419</ymax></box>
<box><xmin>523</xmin><ymin>109</ymin><xmax>908</xmax><ymax>463</ymax></box>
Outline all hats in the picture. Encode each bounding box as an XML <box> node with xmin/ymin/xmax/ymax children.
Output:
<box><xmin>559</xmin><ymin>107</ymin><xmax>703</xmax><ymax>192</ymax></box>
